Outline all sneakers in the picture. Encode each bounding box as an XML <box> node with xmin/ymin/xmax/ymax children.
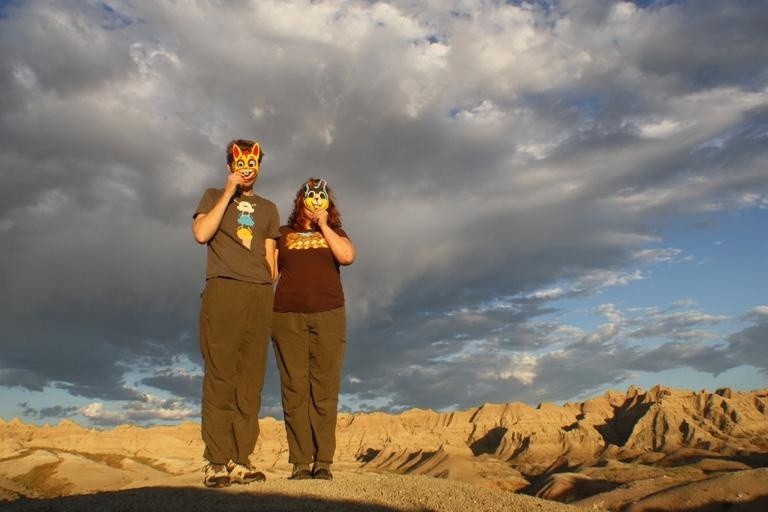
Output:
<box><xmin>312</xmin><ymin>461</ymin><xmax>333</xmax><ymax>481</ymax></box>
<box><xmin>291</xmin><ymin>464</ymin><xmax>312</xmax><ymax>480</ymax></box>
<box><xmin>203</xmin><ymin>463</ymin><xmax>232</xmax><ymax>488</ymax></box>
<box><xmin>228</xmin><ymin>460</ymin><xmax>266</xmax><ymax>484</ymax></box>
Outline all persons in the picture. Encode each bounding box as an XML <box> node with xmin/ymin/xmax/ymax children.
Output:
<box><xmin>270</xmin><ymin>177</ymin><xmax>356</xmax><ymax>481</ymax></box>
<box><xmin>192</xmin><ymin>139</ymin><xmax>282</xmax><ymax>487</ymax></box>
